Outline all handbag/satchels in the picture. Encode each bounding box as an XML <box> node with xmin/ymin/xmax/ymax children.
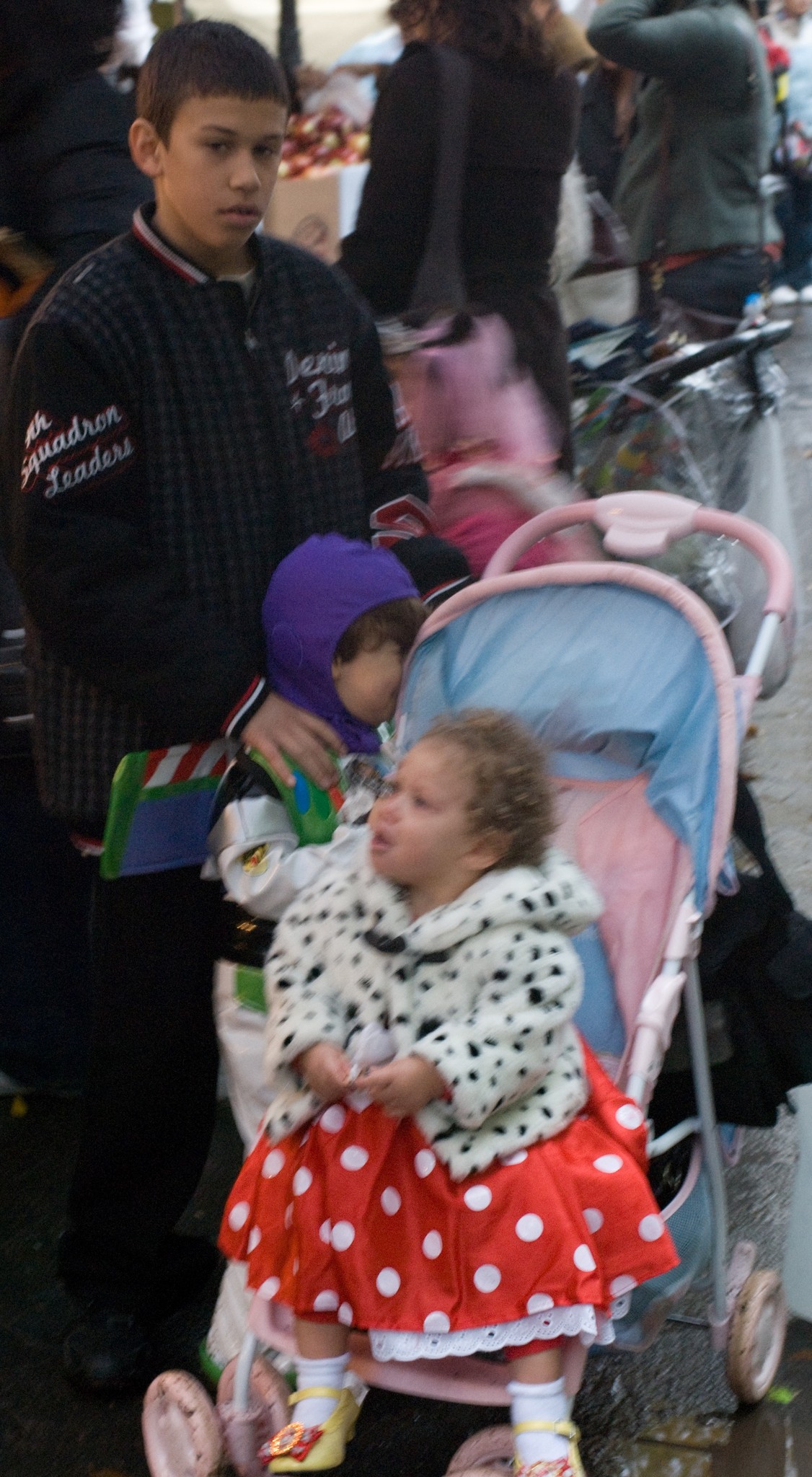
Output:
<box><xmin>378</xmin><ymin>305</ymin><xmax>557</xmax><ymax>510</ymax></box>
<box><xmin>651</xmin><ymin>246</ymin><xmax>779</xmax><ymax>324</ymax></box>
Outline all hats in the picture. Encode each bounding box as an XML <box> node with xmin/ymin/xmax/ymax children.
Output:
<box><xmin>257</xmin><ymin>531</ymin><xmax>420</xmax><ymax>758</ymax></box>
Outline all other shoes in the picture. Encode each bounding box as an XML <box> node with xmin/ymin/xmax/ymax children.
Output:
<box><xmin>258</xmin><ymin>1386</ymin><xmax>360</xmax><ymax>1476</ymax></box>
<box><xmin>768</xmin><ymin>284</ymin><xmax>797</xmax><ymax>306</ymax></box>
<box><xmin>797</xmin><ymin>285</ymin><xmax>812</xmax><ymax>304</ymax></box>
<box><xmin>511</xmin><ymin>1417</ymin><xmax>585</xmax><ymax>1477</ymax></box>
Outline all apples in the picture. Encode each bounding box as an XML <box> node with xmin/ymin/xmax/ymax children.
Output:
<box><xmin>277</xmin><ymin>105</ymin><xmax>371</xmax><ymax>178</ymax></box>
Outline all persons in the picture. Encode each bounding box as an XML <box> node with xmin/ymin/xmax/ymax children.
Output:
<box><xmin>217</xmin><ymin>704</ymin><xmax>686</xmax><ymax>1477</ymax></box>
<box><xmin>0</xmin><ymin>1</ymin><xmax>812</xmax><ymax>1477</ymax></box>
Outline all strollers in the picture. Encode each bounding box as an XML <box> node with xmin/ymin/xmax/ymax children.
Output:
<box><xmin>140</xmin><ymin>302</ymin><xmax>798</xmax><ymax>1477</ymax></box>
